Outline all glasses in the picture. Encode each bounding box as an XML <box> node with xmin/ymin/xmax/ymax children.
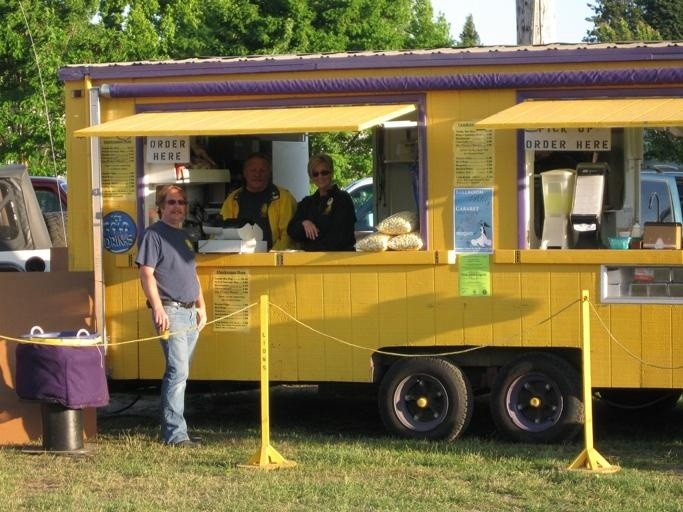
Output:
<box><xmin>309</xmin><ymin>167</ymin><xmax>332</xmax><ymax>178</ymax></box>
<box><xmin>159</xmin><ymin>197</ymin><xmax>188</xmax><ymax>205</ymax></box>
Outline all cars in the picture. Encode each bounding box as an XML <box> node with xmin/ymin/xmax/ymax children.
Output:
<box><xmin>343</xmin><ymin>176</ymin><xmax>376</xmax><ymax>233</ymax></box>
<box><xmin>28</xmin><ymin>174</ymin><xmax>67</xmax><ymax>211</ymax></box>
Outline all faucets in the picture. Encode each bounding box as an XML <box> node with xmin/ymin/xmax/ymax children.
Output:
<box><xmin>648</xmin><ymin>191</ymin><xmax>663</xmax><ymax>223</ymax></box>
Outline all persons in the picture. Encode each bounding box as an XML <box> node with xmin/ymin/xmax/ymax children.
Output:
<box><xmin>133</xmin><ymin>183</ymin><xmax>211</xmax><ymax>451</ymax></box>
<box><xmin>286</xmin><ymin>152</ymin><xmax>358</xmax><ymax>252</ymax></box>
<box><xmin>218</xmin><ymin>154</ymin><xmax>298</xmax><ymax>251</ymax></box>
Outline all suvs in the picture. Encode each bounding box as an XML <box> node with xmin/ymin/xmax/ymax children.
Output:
<box><xmin>639</xmin><ymin>163</ymin><xmax>683</xmax><ymax>226</ymax></box>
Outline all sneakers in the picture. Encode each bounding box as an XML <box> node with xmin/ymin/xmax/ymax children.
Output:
<box><xmin>163</xmin><ymin>433</ymin><xmax>198</xmax><ymax>449</ymax></box>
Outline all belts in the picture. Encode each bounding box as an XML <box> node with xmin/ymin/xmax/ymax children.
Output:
<box><xmin>150</xmin><ymin>294</ymin><xmax>197</xmax><ymax>310</ymax></box>
